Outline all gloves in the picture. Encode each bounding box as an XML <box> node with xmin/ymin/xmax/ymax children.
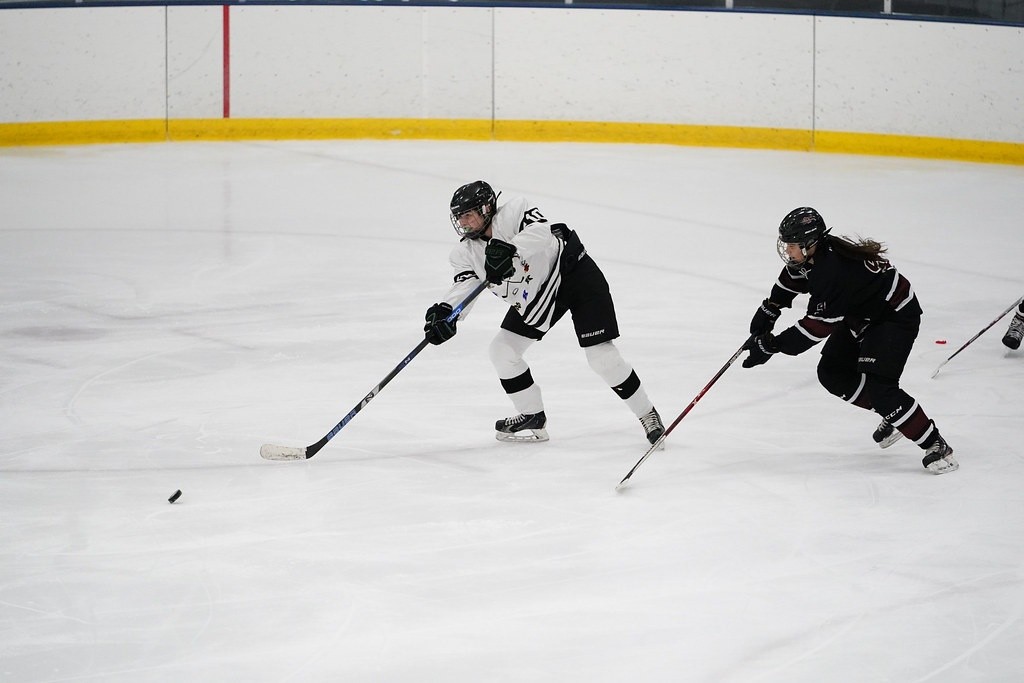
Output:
<box><xmin>742</xmin><ymin>334</ymin><xmax>780</xmax><ymax>368</ymax></box>
<box><xmin>484</xmin><ymin>239</ymin><xmax>517</xmax><ymax>285</ymax></box>
<box><xmin>424</xmin><ymin>302</ymin><xmax>459</xmax><ymax>345</ymax></box>
<box><xmin>750</xmin><ymin>298</ymin><xmax>781</xmax><ymax>336</ymax></box>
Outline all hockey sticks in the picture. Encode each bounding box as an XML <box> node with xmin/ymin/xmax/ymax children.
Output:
<box><xmin>928</xmin><ymin>294</ymin><xmax>1024</xmax><ymax>379</ymax></box>
<box><xmin>614</xmin><ymin>335</ymin><xmax>753</xmax><ymax>491</ymax></box>
<box><xmin>258</xmin><ymin>278</ymin><xmax>491</xmax><ymax>460</ymax></box>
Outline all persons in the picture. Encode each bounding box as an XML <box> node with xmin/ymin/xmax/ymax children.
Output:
<box><xmin>743</xmin><ymin>207</ymin><xmax>953</xmax><ymax>469</ymax></box>
<box><xmin>424</xmin><ymin>180</ymin><xmax>666</xmax><ymax>445</ymax></box>
<box><xmin>1001</xmin><ymin>299</ymin><xmax>1024</xmax><ymax>351</ymax></box>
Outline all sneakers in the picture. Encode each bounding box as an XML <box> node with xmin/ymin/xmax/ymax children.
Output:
<box><xmin>922</xmin><ymin>419</ymin><xmax>959</xmax><ymax>474</ymax></box>
<box><xmin>1002</xmin><ymin>305</ymin><xmax>1024</xmax><ymax>358</ymax></box>
<box><xmin>495</xmin><ymin>410</ymin><xmax>550</xmax><ymax>442</ymax></box>
<box><xmin>639</xmin><ymin>406</ymin><xmax>665</xmax><ymax>449</ymax></box>
<box><xmin>873</xmin><ymin>417</ymin><xmax>904</xmax><ymax>449</ymax></box>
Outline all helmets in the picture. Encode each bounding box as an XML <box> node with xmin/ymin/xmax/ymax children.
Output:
<box><xmin>777</xmin><ymin>207</ymin><xmax>826</xmax><ymax>270</ymax></box>
<box><xmin>449</xmin><ymin>181</ymin><xmax>497</xmax><ymax>240</ymax></box>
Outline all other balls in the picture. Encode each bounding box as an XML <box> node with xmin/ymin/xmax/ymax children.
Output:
<box><xmin>167</xmin><ymin>489</ymin><xmax>183</xmax><ymax>504</ymax></box>
